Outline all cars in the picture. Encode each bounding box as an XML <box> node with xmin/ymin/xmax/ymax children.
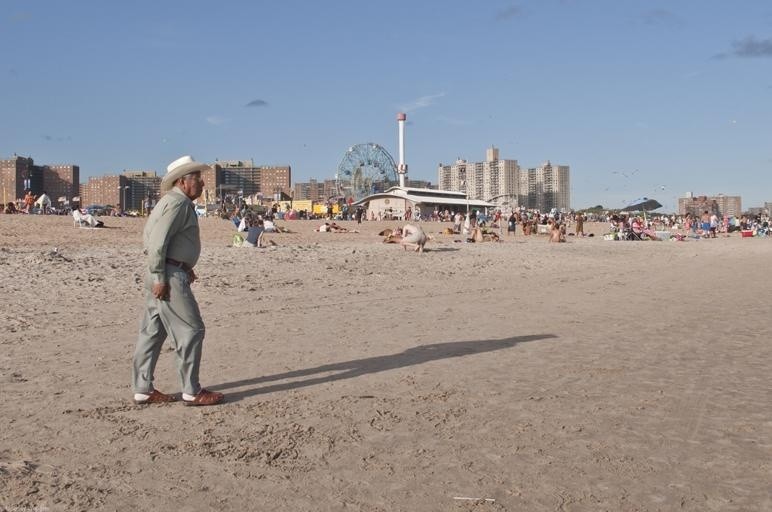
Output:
<box><xmin>83</xmin><ymin>205</ymin><xmax>106</xmax><ymax>216</ymax></box>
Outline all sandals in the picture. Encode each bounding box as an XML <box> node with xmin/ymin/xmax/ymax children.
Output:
<box><xmin>183</xmin><ymin>389</ymin><xmax>224</xmax><ymax>405</ymax></box>
<box><xmin>134</xmin><ymin>388</ymin><xmax>178</xmax><ymax>405</ymax></box>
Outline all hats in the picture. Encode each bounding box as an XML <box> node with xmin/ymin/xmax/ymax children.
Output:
<box><xmin>161</xmin><ymin>155</ymin><xmax>213</xmax><ymax>191</ymax></box>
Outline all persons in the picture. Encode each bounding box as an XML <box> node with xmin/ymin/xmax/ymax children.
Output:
<box><xmin>132</xmin><ymin>155</ymin><xmax>224</xmax><ymax>406</ymax></box>
<box><xmin>73</xmin><ymin>205</ymin><xmax>104</xmax><ymax>227</ymax></box>
<box><xmin>144</xmin><ymin>194</ymin><xmax>152</xmax><ymax>216</ymax></box>
<box><xmin>151</xmin><ymin>197</ymin><xmax>157</xmax><ymax>208</ymax></box>
<box><xmin>372</xmin><ymin>183</ymin><xmax>376</xmax><ymax>194</ymax></box>
<box><xmin>3</xmin><ymin>189</ymin><xmax>126</xmax><ymax>216</ymax></box>
<box><xmin>217</xmin><ymin>195</ymin><xmax>772</xmax><ymax>253</ymax></box>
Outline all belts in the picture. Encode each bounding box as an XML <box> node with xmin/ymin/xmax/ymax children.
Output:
<box><xmin>167</xmin><ymin>258</ymin><xmax>198</xmax><ymax>281</ymax></box>
